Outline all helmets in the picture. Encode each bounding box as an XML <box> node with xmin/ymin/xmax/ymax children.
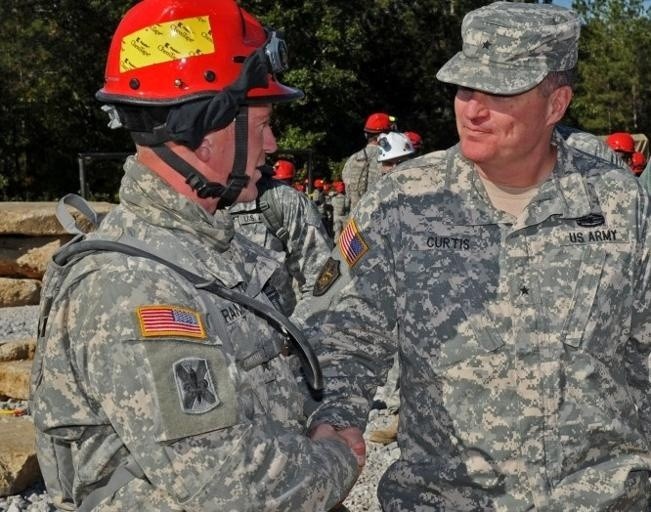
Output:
<box><xmin>629</xmin><ymin>152</ymin><xmax>646</xmax><ymax>173</ymax></box>
<box><xmin>404</xmin><ymin>131</ymin><xmax>423</xmax><ymax>149</ymax></box>
<box><xmin>376</xmin><ymin>131</ymin><xmax>415</xmax><ymax>162</ymax></box>
<box><xmin>271</xmin><ymin>160</ymin><xmax>308</xmax><ymax>192</ymax></box>
<box><xmin>363</xmin><ymin>112</ymin><xmax>391</xmax><ymax>133</ymax></box>
<box><xmin>94</xmin><ymin>0</ymin><xmax>303</xmax><ymax>106</ymax></box>
<box><xmin>607</xmin><ymin>132</ymin><xmax>635</xmax><ymax>153</ymax></box>
<box><xmin>314</xmin><ymin>179</ymin><xmax>344</xmax><ymax>192</ymax></box>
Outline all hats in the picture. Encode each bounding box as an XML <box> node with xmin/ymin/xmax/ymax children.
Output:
<box><xmin>435</xmin><ymin>1</ymin><xmax>582</xmax><ymax>96</ymax></box>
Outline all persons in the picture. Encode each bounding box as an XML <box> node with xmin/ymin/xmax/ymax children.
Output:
<box><xmin>31</xmin><ymin>0</ymin><xmax>368</xmax><ymax>511</ymax></box>
<box><xmin>310</xmin><ymin>1</ymin><xmax>651</xmax><ymax>511</ymax></box>
<box><xmin>556</xmin><ymin>116</ymin><xmax>650</xmax><ymax>190</ymax></box>
<box><xmin>225</xmin><ymin>112</ymin><xmax>423</xmax><ymax>317</ymax></box>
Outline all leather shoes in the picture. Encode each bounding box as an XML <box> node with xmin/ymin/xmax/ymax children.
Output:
<box><xmin>369</xmin><ymin>419</ymin><xmax>399</xmax><ymax>445</ymax></box>
